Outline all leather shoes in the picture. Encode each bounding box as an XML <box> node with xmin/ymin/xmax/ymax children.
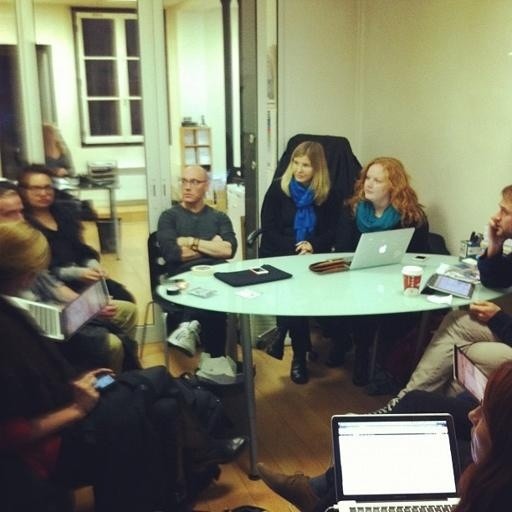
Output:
<box><xmin>200</xmin><ymin>436</ymin><xmax>247</xmax><ymax>464</ymax></box>
<box><xmin>256</xmin><ymin>327</ymin><xmax>285</xmax><ymax>360</ymax></box>
<box><xmin>291</xmin><ymin>359</ymin><xmax>309</xmax><ymax>383</ymax></box>
<box><xmin>256</xmin><ymin>463</ymin><xmax>320</xmax><ymax>512</ymax></box>
<box><xmin>326</xmin><ymin>334</ymin><xmax>352</xmax><ymax>367</ymax></box>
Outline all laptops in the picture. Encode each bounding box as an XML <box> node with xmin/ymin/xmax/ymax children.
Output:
<box><xmin>0</xmin><ymin>277</ymin><xmax>111</xmax><ymax>344</ymax></box>
<box><xmin>453</xmin><ymin>344</ymin><xmax>489</xmax><ymax>404</ymax></box>
<box><xmin>330</xmin><ymin>412</ymin><xmax>462</xmax><ymax>512</ymax></box>
<box><xmin>343</xmin><ymin>227</ymin><xmax>415</xmax><ymax>270</ymax></box>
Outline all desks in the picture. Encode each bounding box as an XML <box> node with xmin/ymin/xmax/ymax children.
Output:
<box><xmin>156</xmin><ymin>252</ymin><xmax>512</xmax><ymax>481</ymax></box>
<box><xmin>79</xmin><ymin>177</ymin><xmax>121</xmax><ymax>260</ymax></box>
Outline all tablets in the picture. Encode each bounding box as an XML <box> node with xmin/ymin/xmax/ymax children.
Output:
<box><xmin>426</xmin><ymin>273</ymin><xmax>475</xmax><ymax>299</ymax></box>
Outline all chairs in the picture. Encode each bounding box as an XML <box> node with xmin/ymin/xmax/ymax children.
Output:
<box><xmin>246</xmin><ymin>134</ymin><xmax>362</xmax><ymax>361</ymax></box>
<box><xmin>140</xmin><ymin>231</ymin><xmax>176</xmax><ymax>369</ymax></box>
<box><xmin>370</xmin><ymin>233</ymin><xmax>451</xmax><ymax>378</ymax></box>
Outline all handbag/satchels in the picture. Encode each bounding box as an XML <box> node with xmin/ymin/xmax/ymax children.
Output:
<box><xmin>175</xmin><ymin>371</ymin><xmax>234</xmax><ymax>439</ymax></box>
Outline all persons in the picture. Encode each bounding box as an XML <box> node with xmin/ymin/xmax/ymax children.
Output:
<box><xmin>261</xmin><ymin>140</ymin><xmax>340</xmax><ymax>384</ymax></box>
<box><xmin>340</xmin><ymin>157</ymin><xmax>430</xmax><ymax>386</ymax></box>
<box><xmin>371</xmin><ymin>186</ymin><xmax>511</xmax><ymax>415</ymax></box>
<box><xmin>256</xmin><ymin>358</ymin><xmax>512</xmax><ymax>512</ymax></box>
<box><xmin>0</xmin><ymin>165</ymin><xmax>246</xmax><ymax>512</ymax></box>
<box><xmin>43</xmin><ymin>125</ymin><xmax>72</xmax><ymax>177</ymax></box>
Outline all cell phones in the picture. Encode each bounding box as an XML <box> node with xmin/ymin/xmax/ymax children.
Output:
<box><xmin>250</xmin><ymin>266</ymin><xmax>269</xmax><ymax>275</ymax></box>
<box><xmin>94</xmin><ymin>374</ymin><xmax>120</xmax><ymax>392</ymax></box>
<box><xmin>414</xmin><ymin>255</ymin><xmax>427</xmax><ymax>260</ymax></box>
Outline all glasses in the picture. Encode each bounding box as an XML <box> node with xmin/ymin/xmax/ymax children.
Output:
<box><xmin>179</xmin><ymin>178</ymin><xmax>206</xmax><ymax>184</ymax></box>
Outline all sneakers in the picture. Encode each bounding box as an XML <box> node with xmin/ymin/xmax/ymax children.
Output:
<box><xmin>167</xmin><ymin>320</ymin><xmax>202</xmax><ymax>357</ymax></box>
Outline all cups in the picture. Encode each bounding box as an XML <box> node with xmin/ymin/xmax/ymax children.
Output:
<box><xmin>401</xmin><ymin>265</ymin><xmax>424</xmax><ymax>298</ymax></box>
